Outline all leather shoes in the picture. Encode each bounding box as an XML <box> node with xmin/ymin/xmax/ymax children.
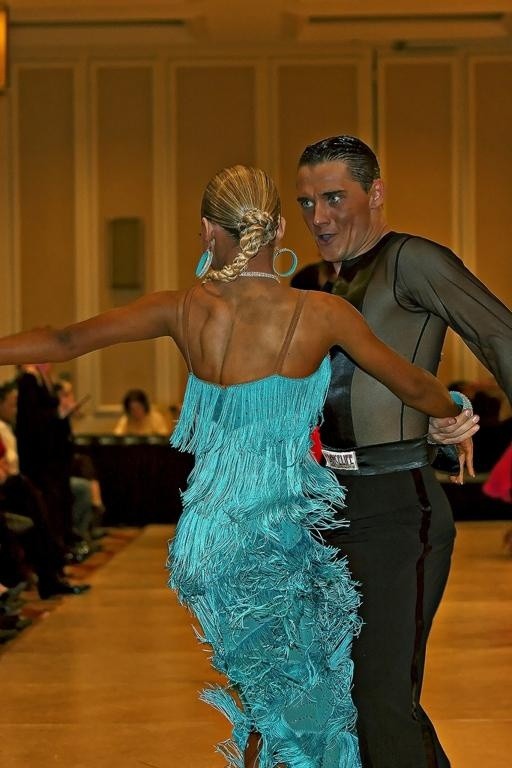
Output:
<box><xmin>61</xmin><ymin>526</ymin><xmax>110</xmax><ymax>565</ymax></box>
<box><xmin>37</xmin><ymin>582</ymin><xmax>91</xmax><ymax>598</ymax></box>
<box><xmin>0</xmin><ymin>575</ymin><xmax>33</xmax><ymax>639</ymax></box>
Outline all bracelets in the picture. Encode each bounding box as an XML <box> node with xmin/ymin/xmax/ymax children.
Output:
<box><xmin>447</xmin><ymin>390</ymin><xmax>474</xmax><ymax>417</ymax></box>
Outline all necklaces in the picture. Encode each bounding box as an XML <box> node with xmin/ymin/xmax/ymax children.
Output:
<box><xmin>238</xmin><ymin>270</ymin><xmax>281</xmax><ymax>284</ymax></box>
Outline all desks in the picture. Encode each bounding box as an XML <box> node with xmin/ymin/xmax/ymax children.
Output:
<box><xmin>72</xmin><ymin>433</ymin><xmax>195</xmax><ymax>530</ymax></box>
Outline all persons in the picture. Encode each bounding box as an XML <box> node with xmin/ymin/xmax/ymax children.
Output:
<box><xmin>112</xmin><ymin>385</ymin><xmax>173</xmax><ymax>438</ymax></box>
<box><xmin>1</xmin><ymin>161</ymin><xmax>480</xmax><ymax>768</ymax></box>
<box><xmin>0</xmin><ymin>353</ymin><xmax>110</xmax><ymax>643</ymax></box>
<box><xmin>285</xmin><ymin>133</ymin><xmax>511</xmax><ymax>767</ymax></box>
<box><xmin>479</xmin><ymin>393</ymin><xmax>512</xmax><ymax>554</ymax></box>
